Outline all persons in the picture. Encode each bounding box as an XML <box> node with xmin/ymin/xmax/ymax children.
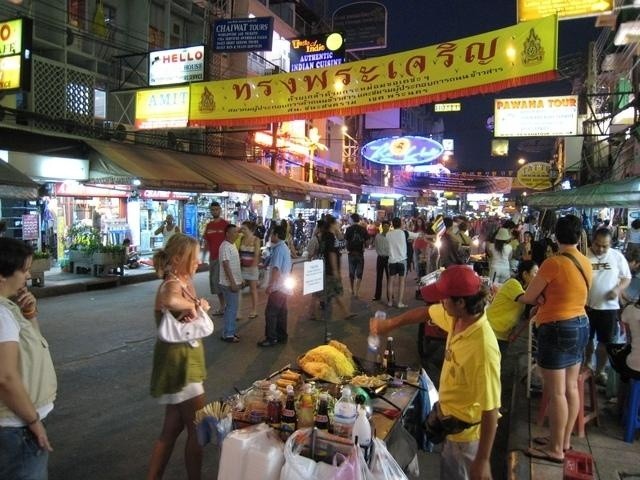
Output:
<box><xmin>121</xmin><ymin>197</ymin><xmax>640</xmax><ymax>383</ymax></box>
<box><xmin>524</xmin><ymin>213</ymin><xmax>592</xmax><ymax>459</ymax></box>
<box><xmin>0</xmin><ymin>237</ymin><xmax>58</xmax><ymax>480</ymax></box>
<box><xmin>146</xmin><ymin>233</ymin><xmax>214</xmax><ymax>480</ymax></box>
<box><xmin>370</xmin><ymin>264</ymin><xmax>503</xmax><ymax>479</ymax></box>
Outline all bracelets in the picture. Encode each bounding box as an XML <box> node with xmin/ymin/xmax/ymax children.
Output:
<box><xmin>26</xmin><ymin>412</ymin><xmax>40</xmax><ymax>425</ymax></box>
<box><xmin>22</xmin><ymin>307</ymin><xmax>39</xmax><ymax>320</ymax></box>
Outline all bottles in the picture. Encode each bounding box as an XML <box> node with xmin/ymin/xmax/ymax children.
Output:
<box><xmin>267</xmin><ymin>384</ymin><xmax>282</xmax><ymax>437</ymax></box>
<box><xmin>282</xmin><ymin>389</ymin><xmax>296</xmax><ymax>440</ymax></box>
<box><xmin>384</xmin><ymin>337</ymin><xmax>395</xmax><ymax>371</ymax></box>
<box><xmin>367</xmin><ymin>309</ymin><xmax>386</xmax><ymax>352</ymax></box>
<box><xmin>296</xmin><ymin>384</ymin><xmax>315</xmax><ymax>454</ymax></box>
<box><xmin>315</xmin><ymin>395</ymin><xmax>330</xmax><ymax>457</ymax></box>
<box><xmin>333</xmin><ymin>387</ymin><xmax>372</xmax><ymax>469</ymax></box>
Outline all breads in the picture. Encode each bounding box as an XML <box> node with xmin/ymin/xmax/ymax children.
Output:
<box><xmin>276</xmin><ymin>370</ymin><xmax>304</xmax><ymax>394</ymax></box>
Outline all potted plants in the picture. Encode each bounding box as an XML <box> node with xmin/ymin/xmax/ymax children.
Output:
<box><xmin>93</xmin><ymin>245</ymin><xmax>125</xmax><ymax>278</ymax></box>
<box><xmin>69</xmin><ymin>237</ymin><xmax>102</xmax><ymax>276</ymax></box>
<box><xmin>29</xmin><ymin>253</ymin><xmax>49</xmax><ymax>287</ymax></box>
<box><xmin>60</xmin><ymin>259</ymin><xmax>72</xmax><ymax>274</ymax></box>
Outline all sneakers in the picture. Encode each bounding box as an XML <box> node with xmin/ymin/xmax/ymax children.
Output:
<box><xmin>220</xmin><ymin>334</ymin><xmax>240</xmax><ymax>343</ymax></box>
<box><xmin>593</xmin><ymin>372</ymin><xmax>607</xmax><ymax>386</ymax></box>
<box><xmin>249</xmin><ymin>313</ymin><xmax>258</xmax><ymax>318</ymax></box>
<box><xmin>212</xmin><ymin>308</ymin><xmax>224</xmax><ymax>315</ymax></box>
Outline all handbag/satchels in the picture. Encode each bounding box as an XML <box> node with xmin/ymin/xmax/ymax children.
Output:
<box><xmin>606</xmin><ymin>343</ymin><xmax>631</xmax><ymax>369</ymax></box>
<box><xmin>423</xmin><ymin>402</ymin><xmax>482</xmax><ymax>444</ymax></box>
<box><xmin>158</xmin><ymin>302</ymin><xmax>214</xmax><ymax>344</ymax></box>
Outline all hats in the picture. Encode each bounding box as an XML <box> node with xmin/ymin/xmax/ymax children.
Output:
<box><xmin>421</xmin><ymin>265</ymin><xmax>481</xmax><ymax>302</ymax></box>
<box><xmin>495</xmin><ymin>228</ymin><xmax>512</xmax><ymax>240</ymax></box>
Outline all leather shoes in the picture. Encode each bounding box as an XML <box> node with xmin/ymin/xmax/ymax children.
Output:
<box><xmin>257</xmin><ymin>337</ymin><xmax>280</xmax><ymax>346</ymax></box>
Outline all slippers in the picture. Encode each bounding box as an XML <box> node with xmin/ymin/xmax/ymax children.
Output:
<box><xmin>524</xmin><ymin>448</ymin><xmax>565</xmax><ymax>463</ymax></box>
<box><xmin>534</xmin><ymin>438</ymin><xmax>572</xmax><ymax>452</ymax></box>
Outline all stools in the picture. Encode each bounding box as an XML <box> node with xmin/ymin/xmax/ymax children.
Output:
<box><xmin>620</xmin><ymin>377</ymin><xmax>640</xmax><ymax>443</ymax></box>
<box><xmin>535</xmin><ymin>366</ymin><xmax>603</xmax><ymax>438</ymax></box>
